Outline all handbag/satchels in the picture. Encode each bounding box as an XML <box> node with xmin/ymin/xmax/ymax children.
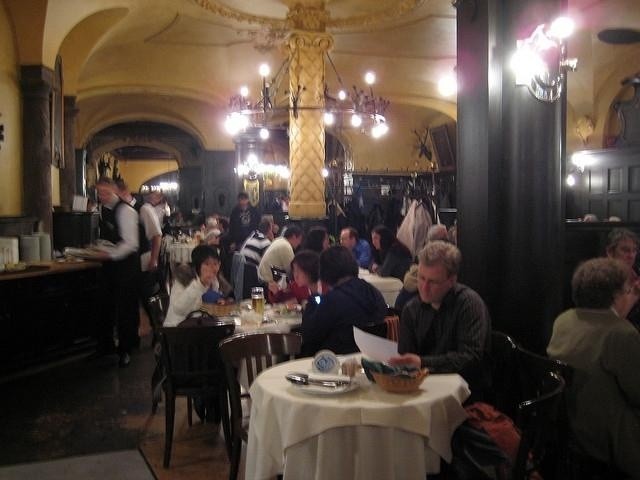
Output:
<box><xmin>171</xmin><ymin>310</ymin><xmax>222</xmax><ymax>388</ymax></box>
<box><xmin>451</xmin><ymin>402</ymin><xmax>541</xmax><ymax>480</ymax></box>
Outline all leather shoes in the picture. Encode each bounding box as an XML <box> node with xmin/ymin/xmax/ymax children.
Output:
<box><xmin>119</xmin><ymin>352</ymin><xmax>129</xmax><ymax>367</ymax></box>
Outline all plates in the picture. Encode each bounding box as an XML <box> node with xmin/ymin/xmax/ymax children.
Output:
<box><xmin>65</xmin><ymin>247</ymin><xmax>107</xmax><ymax>259</ymax></box>
<box><xmin>292</xmin><ymin>374</ymin><xmax>360</xmax><ymax>396</ymax></box>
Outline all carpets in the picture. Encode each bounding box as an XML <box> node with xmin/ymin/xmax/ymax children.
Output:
<box><xmin>1</xmin><ymin>444</ymin><xmax>157</xmax><ymax>479</ymax></box>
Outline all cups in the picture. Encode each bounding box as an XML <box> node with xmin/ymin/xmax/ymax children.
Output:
<box><xmin>251</xmin><ymin>286</ymin><xmax>264</xmax><ymax>316</ymax></box>
<box><xmin>22</xmin><ymin>231</ymin><xmax>52</xmax><ymax>263</ymax></box>
<box><xmin>0</xmin><ymin>238</ymin><xmax>19</xmax><ymax>265</ymax></box>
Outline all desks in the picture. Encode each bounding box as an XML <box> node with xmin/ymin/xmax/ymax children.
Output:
<box><xmin>249</xmin><ymin>355</ymin><xmax>470</xmax><ymax>480</ymax></box>
<box><xmin>168</xmin><ymin>242</ymin><xmax>196</xmax><ymax>264</ymax></box>
<box><xmin>358</xmin><ymin>267</ymin><xmax>402</xmax><ymax>307</ymax></box>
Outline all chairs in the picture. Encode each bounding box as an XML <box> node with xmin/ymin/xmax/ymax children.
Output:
<box><xmin>442</xmin><ymin>333</ymin><xmax>572</xmax><ymax>479</ymax></box>
<box><xmin>359</xmin><ymin>308</ymin><xmax>399</xmax><ymax>338</ymax></box>
<box><xmin>233</xmin><ymin>253</ymin><xmax>260</xmax><ymax>293</ymax></box>
<box><xmin>149</xmin><ymin>293</ymin><xmax>303</xmax><ymax>480</ymax></box>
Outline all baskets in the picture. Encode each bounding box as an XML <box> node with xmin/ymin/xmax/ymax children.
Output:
<box><xmin>205</xmin><ymin>303</ymin><xmax>236</xmax><ymax>317</ymax></box>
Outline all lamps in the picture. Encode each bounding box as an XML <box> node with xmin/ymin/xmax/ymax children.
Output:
<box><xmin>510</xmin><ymin>9</ymin><xmax>585</xmax><ymax>105</ymax></box>
<box><xmin>221</xmin><ymin>45</ymin><xmax>391</xmax><ymax>140</ymax></box>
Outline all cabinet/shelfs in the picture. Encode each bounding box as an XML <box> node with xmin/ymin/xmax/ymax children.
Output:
<box><xmin>53</xmin><ymin>212</ymin><xmax>101</xmax><ymax>250</ymax></box>
